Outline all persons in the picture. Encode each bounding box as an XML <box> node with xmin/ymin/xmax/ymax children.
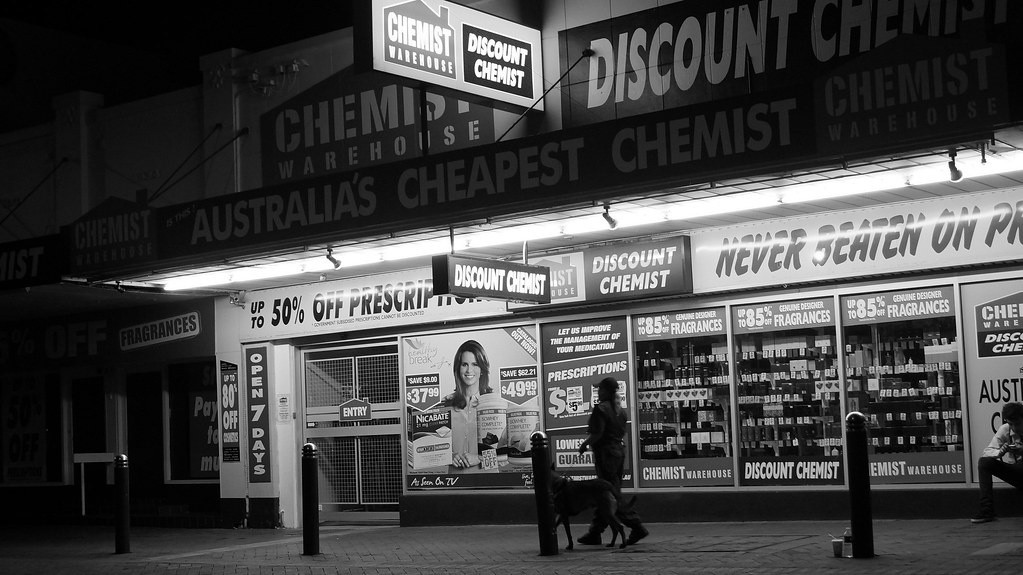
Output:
<box><xmin>428</xmin><ymin>340</ymin><xmax>495</xmax><ymax>468</ymax></box>
<box><xmin>577</xmin><ymin>377</ymin><xmax>649</xmax><ymax>546</ymax></box>
<box><xmin>971</xmin><ymin>402</ymin><xmax>1023</xmax><ymax>522</ymax></box>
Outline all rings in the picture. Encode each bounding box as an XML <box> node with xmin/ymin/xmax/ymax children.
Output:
<box><xmin>458</xmin><ymin>460</ymin><xmax>462</xmax><ymax>463</ymax></box>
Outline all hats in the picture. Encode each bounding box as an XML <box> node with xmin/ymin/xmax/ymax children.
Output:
<box><xmin>593</xmin><ymin>377</ymin><xmax>618</xmax><ymax>393</ymax></box>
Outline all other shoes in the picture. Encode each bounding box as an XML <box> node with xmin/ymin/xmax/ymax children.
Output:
<box><xmin>577</xmin><ymin>530</ymin><xmax>602</xmax><ymax>545</ymax></box>
<box><xmin>627</xmin><ymin>525</ymin><xmax>649</xmax><ymax>545</ymax></box>
<box><xmin>970</xmin><ymin>511</ymin><xmax>994</xmax><ymax>523</ymax></box>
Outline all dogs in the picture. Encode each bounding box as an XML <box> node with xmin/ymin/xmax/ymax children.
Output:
<box><xmin>550</xmin><ymin>462</ymin><xmax>637</xmax><ymax>550</ymax></box>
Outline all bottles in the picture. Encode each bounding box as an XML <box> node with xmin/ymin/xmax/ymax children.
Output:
<box><xmin>505</xmin><ymin>406</ymin><xmax>539</xmax><ymax>464</ymax></box>
<box><xmin>476</xmin><ymin>393</ymin><xmax>508</xmax><ymax>463</ymax></box>
